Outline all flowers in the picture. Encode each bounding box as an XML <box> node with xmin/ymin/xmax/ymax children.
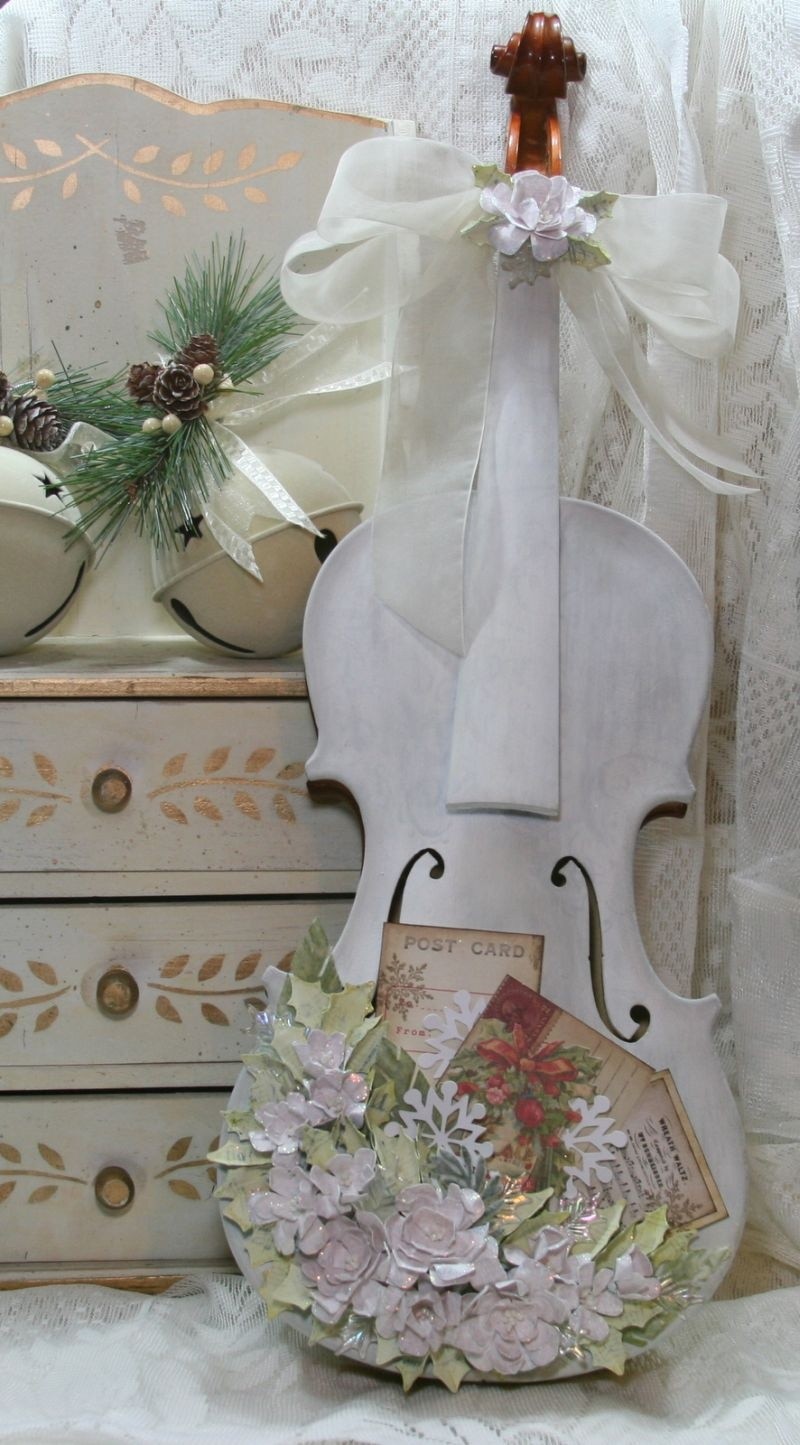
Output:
<box><xmin>208</xmin><ymin>916</ymin><xmax>734</xmax><ymax>1396</ymax></box>
<box><xmin>460</xmin><ymin>164</ymin><xmax>620</xmax><ymax>288</ymax></box>
<box><xmin>0</xmin><ymin>224</ymin><xmax>323</xmax><ymax>568</ymax></box>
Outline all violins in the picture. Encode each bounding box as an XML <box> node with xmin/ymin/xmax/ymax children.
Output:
<box><xmin>210</xmin><ymin>11</ymin><xmax>749</xmax><ymax>1395</ymax></box>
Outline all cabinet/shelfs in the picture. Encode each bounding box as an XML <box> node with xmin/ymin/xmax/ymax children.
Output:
<box><xmin>0</xmin><ymin>73</ymin><xmax>365</xmax><ymax>1297</ymax></box>
